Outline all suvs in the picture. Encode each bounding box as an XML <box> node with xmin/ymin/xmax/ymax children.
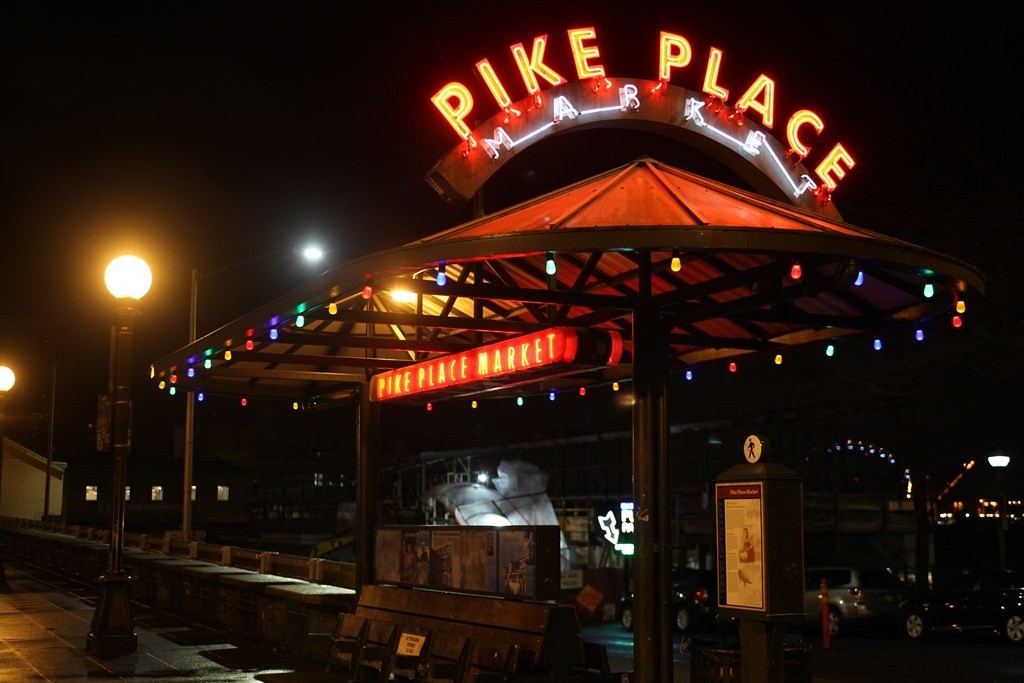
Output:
<box><xmin>803</xmin><ymin>563</ymin><xmax>892</xmax><ymax>638</ymax></box>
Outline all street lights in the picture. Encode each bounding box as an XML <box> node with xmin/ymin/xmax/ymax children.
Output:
<box><xmin>0</xmin><ymin>362</ymin><xmax>17</xmax><ymax>478</ymax></box>
<box><xmin>986</xmin><ymin>450</ymin><xmax>1014</xmax><ymax>571</ymax></box>
<box><xmin>85</xmin><ymin>252</ymin><xmax>152</xmax><ymax>657</ymax></box>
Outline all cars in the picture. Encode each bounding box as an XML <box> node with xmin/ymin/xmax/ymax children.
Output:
<box><xmin>896</xmin><ymin>564</ymin><xmax>1024</xmax><ymax>646</ymax></box>
<box><xmin>619</xmin><ymin>569</ymin><xmax>719</xmax><ymax>637</ymax></box>
